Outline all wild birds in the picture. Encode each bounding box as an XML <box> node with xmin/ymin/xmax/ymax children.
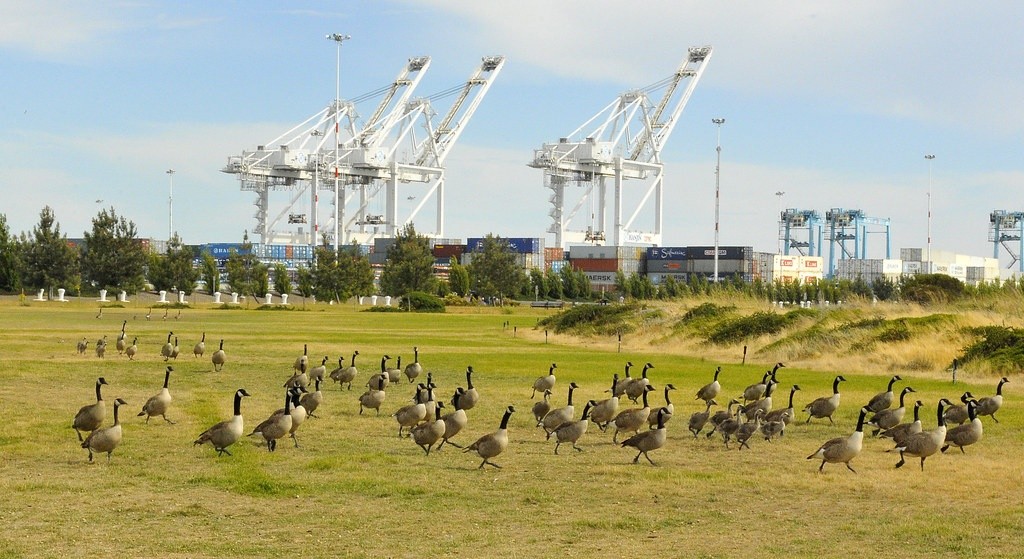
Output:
<box><xmin>192</xmin><ymin>388</ymin><xmax>251</xmax><ymax>458</ymax></box>
<box><xmin>174</xmin><ymin>310</ymin><xmax>181</xmax><ymax>321</ymax></box>
<box><xmin>160</xmin><ymin>336</ymin><xmax>179</xmax><ymax>361</ymax></box>
<box><xmin>77</xmin><ymin>337</ymin><xmax>90</xmax><ymax>354</ymax></box>
<box><xmin>136</xmin><ymin>365</ymin><xmax>177</xmax><ymax>425</ymax></box>
<box><xmin>72</xmin><ymin>377</ymin><xmax>109</xmax><ymax>441</ymax></box>
<box><xmin>145</xmin><ymin>307</ymin><xmax>152</xmax><ymax>321</ymax></box>
<box><xmin>95</xmin><ymin>334</ymin><xmax>108</xmax><ymax>358</ymax></box>
<box><xmin>161</xmin><ymin>331</ymin><xmax>174</xmax><ymax>363</ymax></box>
<box><xmin>116</xmin><ymin>329</ymin><xmax>126</xmax><ymax>354</ymax></box>
<box><xmin>117</xmin><ymin>320</ymin><xmax>128</xmax><ymax>342</ymax></box>
<box><xmin>161</xmin><ymin>308</ymin><xmax>169</xmax><ymax>321</ymax></box>
<box><xmin>211</xmin><ymin>339</ymin><xmax>226</xmax><ymax>371</ymax></box>
<box><xmin>194</xmin><ymin>331</ymin><xmax>207</xmax><ymax>357</ymax></box>
<box><xmin>80</xmin><ymin>397</ymin><xmax>127</xmax><ymax>463</ymax></box>
<box><xmin>96</xmin><ymin>307</ymin><xmax>103</xmax><ymax>320</ymax></box>
<box><xmin>246</xmin><ymin>343</ymin><xmax>1011</xmax><ymax>471</ymax></box>
<box><xmin>126</xmin><ymin>337</ymin><xmax>138</xmax><ymax>362</ymax></box>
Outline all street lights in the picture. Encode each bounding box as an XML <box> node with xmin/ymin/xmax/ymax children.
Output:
<box><xmin>776</xmin><ymin>191</ymin><xmax>785</xmax><ymax>211</ymax></box>
<box><xmin>95</xmin><ymin>199</ymin><xmax>105</xmax><ymax>213</ymax></box>
<box><xmin>925</xmin><ymin>154</ymin><xmax>936</xmax><ymax>274</ymax></box>
<box><xmin>408</xmin><ymin>196</ymin><xmax>415</xmax><ymax>216</ymax></box>
<box><xmin>713</xmin><ymin>118</ymin><xmax>727</xmax><ymax>284</ymax></box>
<box><xmin>167</xmin><ymin>169</ymin><xmax>176</xmax><ymax>239</ymax></box>
<box><xmin>324</xmin><ymin>32</ymin><xmax>352</xmax><ymax>267</ymax></box>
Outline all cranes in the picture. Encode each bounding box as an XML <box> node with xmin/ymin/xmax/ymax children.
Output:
<box><xmin>525</xmin><ymin>46</ymin><xmax>714</xmax><ymax>244</ymax></box>
<box><xmin>220</xmin><ymin>53</ymin><xmax>505</xmax><ymax>277</ymax></box>
